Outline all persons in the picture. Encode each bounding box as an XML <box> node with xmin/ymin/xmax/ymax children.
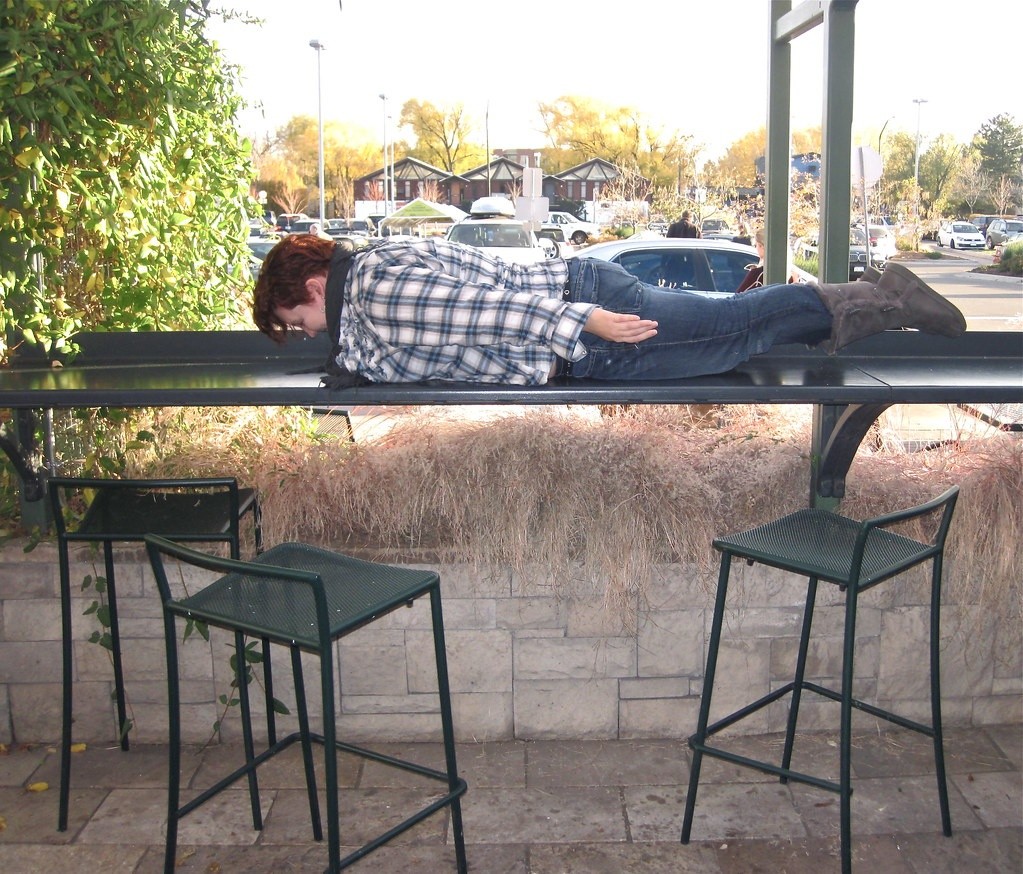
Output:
<box><xmin>656</xmin><ymin>252</ymin><xmax>684</xmax><ymax>290</ymax></box>
<box><xmin>514</xmin><ymin>232</ymin><xmax>526</xmax><ymax>245</ymax></box>
<box><xmin>467</xmin><ymin>232</ymin><xmax>484</xmax><ymax>246</ymax></box>
<box><xmin>733</xmin><ymin>225</ymin><xmax>751</xmax><ymax>246</ymax></box>
<box><xmin>667</xmin><ymin>209</ymin><xmax>703</xmax><ymax>238</ymax></box>
<box><xmin>810</xmin><ymin>240</ymin><xmax>817</xmax><ymax>256</ymax></box>
<box><xmin>251</xmin><ymin>224</ymin><xmax>967</xmax><ymax>387</ymax></box>
<box><xmin>736</xmin><ymin>227</ymin><xmax>798</xmax><ymax>293</ymax></box>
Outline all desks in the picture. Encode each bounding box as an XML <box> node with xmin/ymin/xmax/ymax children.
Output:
<box><xmin>0</xmin><ymin>330</ymin><xmax>1023</xmax><ymax>536</ymax></box>
<box><xmin>957</xmin><ymin>403</ymin><xmax>1023</xmax><ymax>432</ymax></box>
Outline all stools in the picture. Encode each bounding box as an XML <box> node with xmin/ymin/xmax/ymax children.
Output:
<box><xmin>47</xmin><ymin>473</ymin><xmax>265</xmax><ymax>833</ymax></box>
<box><xmin>677</xmin><ymin>481</ymin><xmax>963</xmax><ymax>874</ymax></box>
<box><xmin>143</xmin><ymin>532</ymin><xmax>469</xmax><ymax>874</ymax></box>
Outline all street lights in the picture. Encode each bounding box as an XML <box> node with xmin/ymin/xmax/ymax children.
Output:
<box><xmin>911</xmin><ymin>98</ymin><xmax>928</xmax><ymax>184</ymax></box>
<box><xmin>306</xmin><ymin>38</ymin><xmax>328</xmax><ymax>229</ymax></box>
<box><xmin>378</xmin><ymin>92</ymin><xmax>398</xmax><ymax>219</ymax></box>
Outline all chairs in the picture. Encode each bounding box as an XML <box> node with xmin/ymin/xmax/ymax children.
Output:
<box><xmin>463</xmin><ymin>231</ymin><xmax>484</xmax><ymax>247</ymax></box>
<box><xmin>867</xmin><ymin>418</ymin><xmax>965</xmax><ymax>454</ymax></box>
<box><xmin>502</xmin><ymin>231</ymin><xmax>527</xmax><ymax>246</ymax></box>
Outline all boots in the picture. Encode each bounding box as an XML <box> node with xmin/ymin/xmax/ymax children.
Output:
<box><xmin>792</xmin><ymin>264</ymin><xmax>909</xmax><ymax>352</ymax></box>
<box><xmin>806</xmin><ymin>261</ymin><xmax>968</xmax><ymax>356</ymax></box>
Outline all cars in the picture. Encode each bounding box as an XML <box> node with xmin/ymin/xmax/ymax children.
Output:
<box><xmin>1002</xmin><ymin>232</ymin><xmax>1023</xmax><ymax>247</ymax></box>
<box><xmin>793</xmin><ymin>225</ymin><xmax>888</xmax><ymax>279</ymax></box>
<box><xmin>237</xmin><ymin>197</ymin><xmax>752</xmax><ymax>278</ymax></box>
<box><xmin>854</xmin><ymin>213</ymin><xmax>1011</xmax><ymax>237</ymax></box>
<box><xmin>986</xmin><ymin>219</ymin><xmax>1023</xmax><ymax>249</ymax></box>
<box><xmin>936</xmin><ymin>219</ymin><xmax>987</xmax><ymax>250</ymax></box>
<box><xmin>564</xmin><ymin>238</ymin><xmax>821</xmax><ymax>300</ymax></box>
